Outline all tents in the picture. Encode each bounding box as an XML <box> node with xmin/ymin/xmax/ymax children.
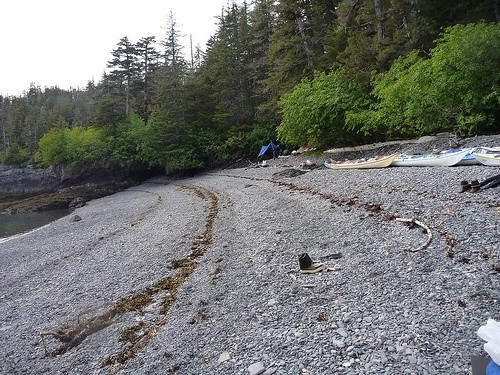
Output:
<box><xmin>256</xmin><ymin>140</ymin><xmax>281</xmax><ymax>162</ymax></box>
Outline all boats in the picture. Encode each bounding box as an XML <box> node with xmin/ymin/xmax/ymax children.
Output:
<box><xmin>455</xmin><ymin>153</ymin><xmax>479</xmax><ymax>165</ymax></box>
<box><xmin>324</xmin><ymin>154</ymin><xmax>396</xmax><ymax>168</ymax></box>
<box><xmin>391</xmin><ymin>149</ymin><xmax>470</xmax><ymax>166</ymax></box>
<box><xmin>472</xmin><ymin>152</ymin><xmax>500</xmax><ymax>166</ymax></box>
